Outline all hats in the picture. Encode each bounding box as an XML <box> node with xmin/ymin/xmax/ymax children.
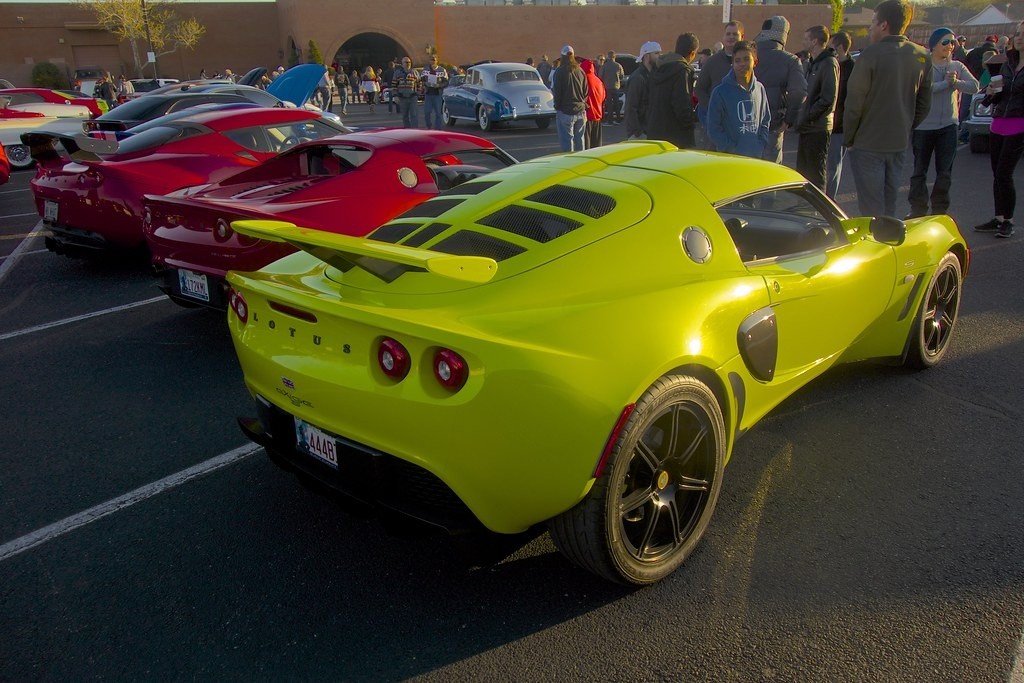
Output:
<box><xmin>752</xmin><ymin>16</ymin><xmax>789</xmax><ymax>46</ymax></box>
<box><xmin>278</xmin><ymin>66</ymin><xmax>284</xmax><ymax>70</ymax></box>
<box><xmin>636</xmin><ymin>41</ymin><xmax>661</xmax><ymax>62</ymax></box>
<box><xmin>928</xmin><ymin>27</ymin><xmax>955</xmax><ymax>52</ymax></box>
<box><xmin>561</xmin><ymin>46</ymin><xmax>574</xmax><ymax>56</ymax></box>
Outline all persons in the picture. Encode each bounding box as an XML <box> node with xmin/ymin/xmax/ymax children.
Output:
<box><xmin>200</xmin><ymin>65</ymin><xmax>284</xmax><ymax>91</ymax></box>
<box><xmin>391</xmin><ymin>57</ymin><xmax>422</xmax><ymax>128</ymax></box>
<box><xmin>951</xmin><ymin>34</ymin><xmax>1014</xmax><ymax>145</ymax></box>
<box><xmin>310</xmin><ymin>57</ymin><xmax>402</xmax><ymax>117</ymax></box>
<box><xmin>526</xmin><ymin>45</ymin><xmax>624</xmax><ymax>152</ymax></box>
<box><xmin>94</xmin><ymin>73</ymin><xmax>135</xmax><ymax>109</ymax></box>
<box><xmin>904</xmin><ymin>27</ymin><xmax>981</xmax><ymax>219</ymax></box>
<box><xmin>842</xmin><ymin>0</ymin><xmax>933</xmax><ymax>220</ymax></box>
<box><xmin>421</xmin><ymin>54</ymin><xmax>448</xmax><ymax>129</ymax></box>
<box><xmin>973</xmin><ymin>21</ymin><xmax>1024</xmax><ymax>239</ymax></box>
<box><xmin>623</xmin><ymin>15</ymin><xmax>856</xmax><ymax>214</ymax></box>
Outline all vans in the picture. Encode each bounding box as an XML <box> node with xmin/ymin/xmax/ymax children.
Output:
<box><xmin>127</xmin><ymin>79</ymin><xmax>180</xmax><ymax>96</ymax></box>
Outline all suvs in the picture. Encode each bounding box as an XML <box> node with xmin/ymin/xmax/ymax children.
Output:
<box><xmin>70</xmin><ymin>66</ymin><xmax>108</xmax><ymax>98</ymax></box>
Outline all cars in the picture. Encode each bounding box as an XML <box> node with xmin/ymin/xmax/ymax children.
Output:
<box><xmin>88</xmin><ymin>94</ymin><xmax>254</xmax><ymax>142</ymax></box>
<box><xmin>555</xmin><ymin>55</ymin><xmax>586</xmax><ymax>66</ymax></box>
<box><xmin>961</xmin><ymin>54</ymin><xmax>1008</xmax><ymax>152</ymax></box>
<box><xmin>145</xmin><ymin>61</ymin><xmax>344</xmax><ymax>133</ymax></box>
<box><xmin>379</xmin><ymin>67</ymin><xmax>427</xmax><ymax>105</ymax></box>
<box><xmin>439</xmin><ymin>63</ymin><xmax>554</xmax><ymax>132</ymax></box>
<box><xmin>0</xmin><ymin>79</ymin><xmax>109</xmax><ymax>170</ymax></box>
<box><xmin>615</xmin><ymin>54</ymin><xmax>642</xmax><ymax>85</ymax></box>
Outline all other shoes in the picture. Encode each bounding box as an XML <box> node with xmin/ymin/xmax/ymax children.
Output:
<box><xmin>602</xmin><ymin>122</ymin><xmax>613</xmax><ymax>126</ymax></box>
<box><xmin>613</xmin><ymin>121</ymin><xmax>620</xmax><ymax>124</ymax></box>
<box><xmin>957</xmin><ymin>133</ymin><xmax>969</xmax><ymax>145</ymax></box>
<box><xmin>904</xmin><ymin>212</ymin><xmax>918</xmax><ymax>220</ymax></box>
<box><xmin>396</xmin><ymin>110</ymin><xmax>400</xmax><ymax>113</ymax></box>
<box><xmin>389</xmin><ymin>111</ymin><xmax>392</xmax><ymax>113</ymax></box>
<box><xmin>341</xmin><ymin>110</ymin><xmax>348</xmax><ymax>116</ymax></box>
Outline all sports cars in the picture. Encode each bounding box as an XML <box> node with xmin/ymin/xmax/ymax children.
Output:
<box><xmin>20</xmin><ymin>106</ymin><xmax>405</xmax><ymax>275</ymax></box>
<box><xmin>141</xmin><ymin>123</ymin><xmax>523</xmax><ymax>314</ymax></box>
<box><xmin>225</xmin><ymin>139</ymin><xmax>970</xmax><ymax>586</ymax></box>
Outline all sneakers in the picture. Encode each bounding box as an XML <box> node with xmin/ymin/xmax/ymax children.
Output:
<box><xmin>995</xmin><ymin>220</ymin><xmax>1015</xmax><ymax>238</ymax></box>
<box><xmin>973</xmin><ymin>217</ymin><xmax>1002</xmax><ymax>231</ymax></box>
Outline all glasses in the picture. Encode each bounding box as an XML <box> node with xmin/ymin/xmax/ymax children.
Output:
<box><xmin>963</xmin><ymin>40</ymin><xmax>966</xmax><ymax>42</ymax></box>
<box><xmin>941</xmin><ymin>38</ymin><xmax>956</xmax><ymax>45</ymax></box>
<box><xmin>406</xmin><ymin>61</ymin><xmax>411</xmax><ymax>63</ymax></box>
<box><xmin>600</xmin><ymin>59</ymin><xmax>605</xmax><ymax>62</ymax></box>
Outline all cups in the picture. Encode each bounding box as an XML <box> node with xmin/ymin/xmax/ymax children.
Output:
<box><xmin>991</xmin><ymin>75</ymin><xmax>1003</xmax><ymax>94</ymax></box>
